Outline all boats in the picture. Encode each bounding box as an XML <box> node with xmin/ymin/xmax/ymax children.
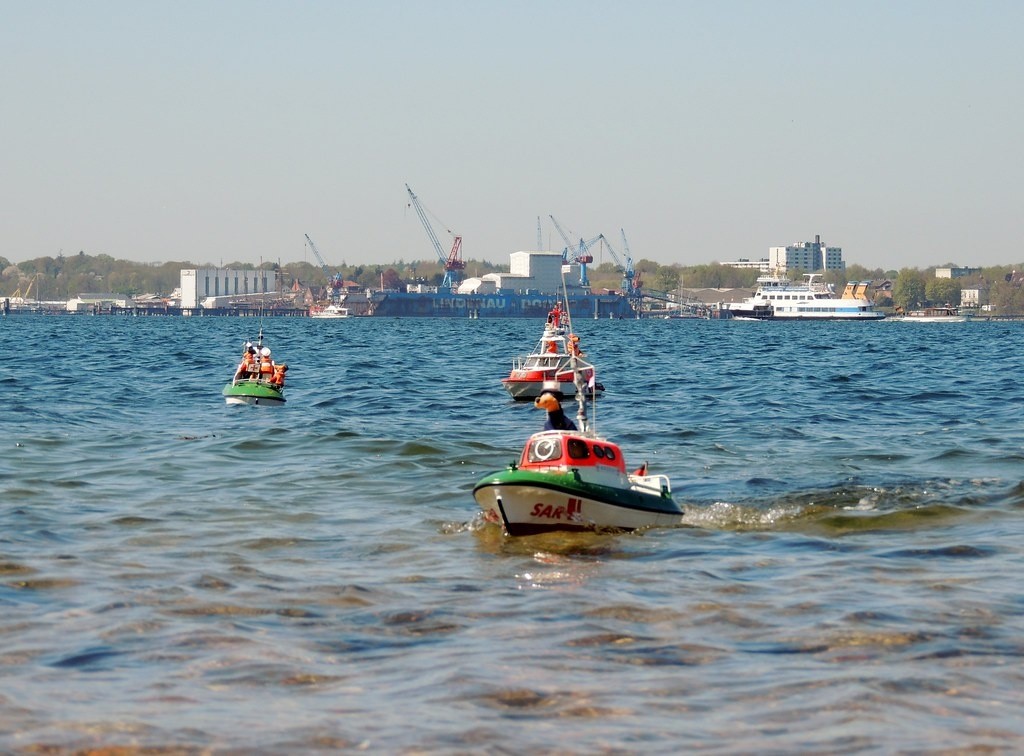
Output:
<box><xmin>312</xmin><ymin>302</ymin><xmax>355</xmax><ymax>319</ymax></box>
<box><xmin>500</xmin><ymin>284</ymin><xmax>603</xmax><ymax>400</ymax></box>
<box><xmin>718</xmin><ymin>248</ymin><xmax>886</xmax><ymax>321</ymax></box>
<box><xmin>473</xmin><ymin>270</ymin><xmax>685</xmax><ymax>537</ymax></box>
<box><xmin>222</xmin><ymin>299</ymin><xmax>287</xmax><ymax>407</ymax></box>
